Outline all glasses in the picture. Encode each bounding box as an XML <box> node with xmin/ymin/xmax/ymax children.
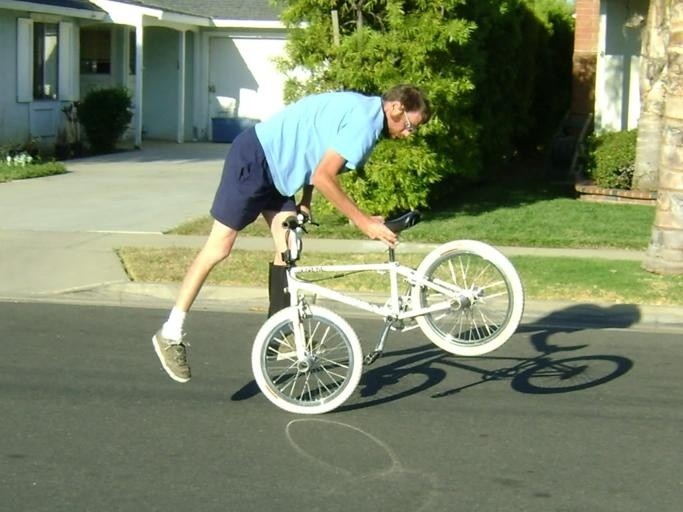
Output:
<box><xmin>403</xmin><ymin>108</ymin><xmax>418</xmax><ymax>132</ymax></box>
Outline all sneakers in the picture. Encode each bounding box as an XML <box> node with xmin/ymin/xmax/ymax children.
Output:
<box><xmin>266</xmin><ymin>332</ymin><xmax>327</xmax><ymax>360</ymax></box>
<box><xmin>152</xmin><ymin>327</ymin><xmax>191</xmax><ymax>384</ymax></box>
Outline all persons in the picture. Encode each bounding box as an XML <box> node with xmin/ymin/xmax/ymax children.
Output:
<box><xmin>151</xmin><ymin>84</ymin><xmax>432</xmax><ymax>384</ymax></box>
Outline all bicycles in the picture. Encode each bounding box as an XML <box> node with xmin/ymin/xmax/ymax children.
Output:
<box><xmin>250</xmin><ymin>211</ymin><xmax>523</xmax><ymax>414</ymax></box>
